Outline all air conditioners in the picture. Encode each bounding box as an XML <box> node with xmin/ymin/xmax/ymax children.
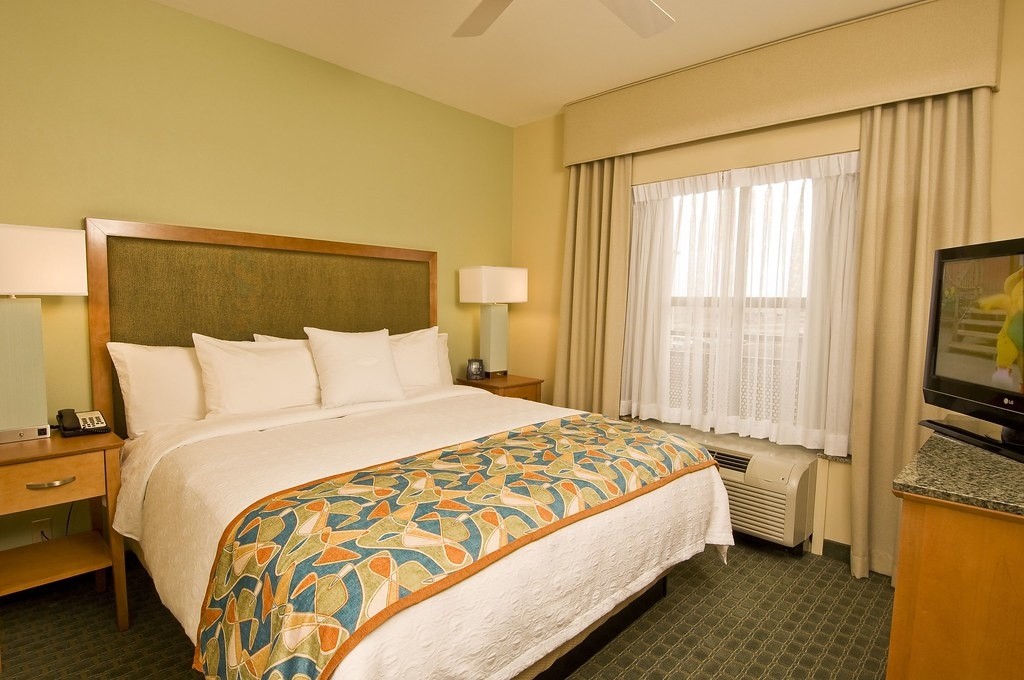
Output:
<box><xmin>640</xmin><ymin>421</ymin><xmax>818</xmax><ymax>547</ymax></box>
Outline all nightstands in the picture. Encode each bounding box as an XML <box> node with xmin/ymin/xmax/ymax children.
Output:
<box><xmin>0</xmin><ymin>430</ymin><xmax>129</xmax><ymax>634</ymax></box>
<box><xmin>457</xmin><ymin>373</ymin><xmax>545</xmax><ymax>403</ymax></box>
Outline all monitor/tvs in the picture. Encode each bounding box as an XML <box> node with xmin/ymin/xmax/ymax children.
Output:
<box><xmin>918</xmin><ymin>236</ymin><xmax>1024</xmax><ymax>464</ymax></box>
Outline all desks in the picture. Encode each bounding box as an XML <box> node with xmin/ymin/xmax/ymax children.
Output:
<box><xmin>886</xmin><ymin>431</ymin><xmax>1024</xmax><ymax>680</ymax></box>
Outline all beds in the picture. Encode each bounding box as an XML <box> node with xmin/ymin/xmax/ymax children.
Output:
<box><xmin>85</xmin><ymin>219</ymin><xmax>734</xmax><ymax>680</ymax></box>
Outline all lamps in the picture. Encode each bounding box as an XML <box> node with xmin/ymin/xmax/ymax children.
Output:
<box><xmin>0</xmin><ymin>224</ymin><xmax>88</xmax><ymax>445</ymax></box>
<box><xmin>458</xmin><ymin>267</ymin><xmax>528</xmax><ymax>378</ymax></box>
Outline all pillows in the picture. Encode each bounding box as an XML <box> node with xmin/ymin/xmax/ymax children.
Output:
<box><xmin>106</xmin><ymin>342</ymin><xmax>206</xmax><ymax>438</ymax></box>
<box><xmin>192</xmin><ymin>332</ymin><xmax>323</xmax><ymax>419</ymax></box>
<box><xmin>389</xmin><ymin>325</ymin><xmax>439</xmax><ymax>391</ymax></box>
<box><xmin>253</xmin><ymin>334</ymin><xmax>292</xmax><ymax>342</ymax></box>
<box><xmin>303</xmin><ymin>326</ymin><xmax>404</xmax><ymax>409</ymax></box>
<box><xmin>439</xmin><ymin>333</ymin><xmax>453</xmax><ymax>389</ymax></box>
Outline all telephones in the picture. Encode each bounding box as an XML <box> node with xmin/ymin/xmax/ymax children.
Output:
<box><xmin>55</xmin><ymin>408</ymin><xmax>111</xmax><ymax>439</ymax></box>
